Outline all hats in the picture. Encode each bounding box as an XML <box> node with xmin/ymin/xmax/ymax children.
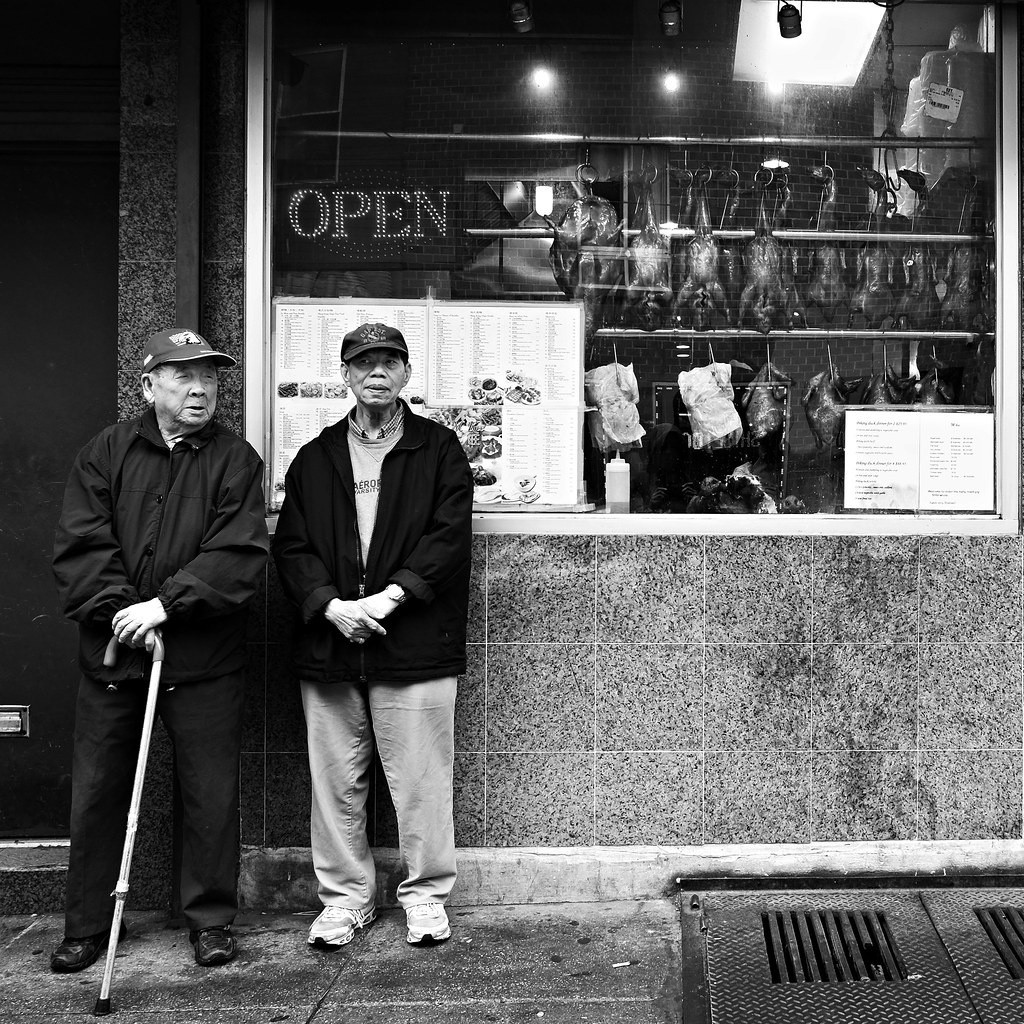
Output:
<box><xmin>341</xmin><ymin>323</ymin><xmax>409</xmax><ymax>364</ymax></box>
<box><xmin>141</xmin><ymin>328</ymin><xmax>237</xmax><ymax>374</ymax></box>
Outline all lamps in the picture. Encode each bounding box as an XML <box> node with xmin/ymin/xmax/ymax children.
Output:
<box><xmin>512</xmin><ymin>3</ymin><xmax>534</xmax><ymax>33</ymax></box>
<box><xmin>657</xmin><ymin>0</ymin><xmax>683</xmax><ymax>36</ymax></box>
<box><xmin>776</xmin><ymin>0</ymin><xmax>802</xmax><ymax>38</ymax></box>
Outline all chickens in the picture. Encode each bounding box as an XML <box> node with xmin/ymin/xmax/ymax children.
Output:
<box><xmin>742</xmin><ymin>362</ymin><xmax>954</xmax><ymax>449</ymax></box>
<box><xmin>545</xmin><ymin>194</ymin><xmax>627</xmax><ymax>354</ymax></box>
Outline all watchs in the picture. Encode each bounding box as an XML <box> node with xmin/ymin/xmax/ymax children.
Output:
<box><xmin>383</xmin><ymin>583</ymin><xmax>407</xmax><ymax>603</ymax></box>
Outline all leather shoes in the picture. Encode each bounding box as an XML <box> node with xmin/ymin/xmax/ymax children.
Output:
<box><xmin>187</xmin><ymin>924</ymin><xmax>237</xmax><ymax>966</ymax></box>
<box><xmin>50</xmin><ymin>921</ymin><xmax>129</xmax><ymax>972</ymax></box>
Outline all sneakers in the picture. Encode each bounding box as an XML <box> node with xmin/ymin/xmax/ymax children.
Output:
<box><xmin>306</xmin><ymin>903</ymin><xmax>377</xmax><ymax>946</ymax></box>
<box><xmin>406</xmin><ymin>902</ymin><xmax>450</xmax><ymax>944</ymax></box>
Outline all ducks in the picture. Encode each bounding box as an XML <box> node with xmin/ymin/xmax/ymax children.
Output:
<box><xmin>613</xmin><ymin>163</ymin><xmax>995</xmax><ymax>331</ymax></box>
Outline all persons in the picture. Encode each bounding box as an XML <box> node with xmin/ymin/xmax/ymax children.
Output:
<box><xmin>50</xmin><ymin>329</ymin><xmax>269</xmax><ymax>975</ymax></box>
<box><xmin>271</xmin><ymin>323</ymin><xmax>474</xmax><ymax>947</ymax></box>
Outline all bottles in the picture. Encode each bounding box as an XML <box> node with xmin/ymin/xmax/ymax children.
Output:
<box><xmin>606</xmin><ymin>449</ymin><xmax>630</xmax><ymax>514</ymax></box>
